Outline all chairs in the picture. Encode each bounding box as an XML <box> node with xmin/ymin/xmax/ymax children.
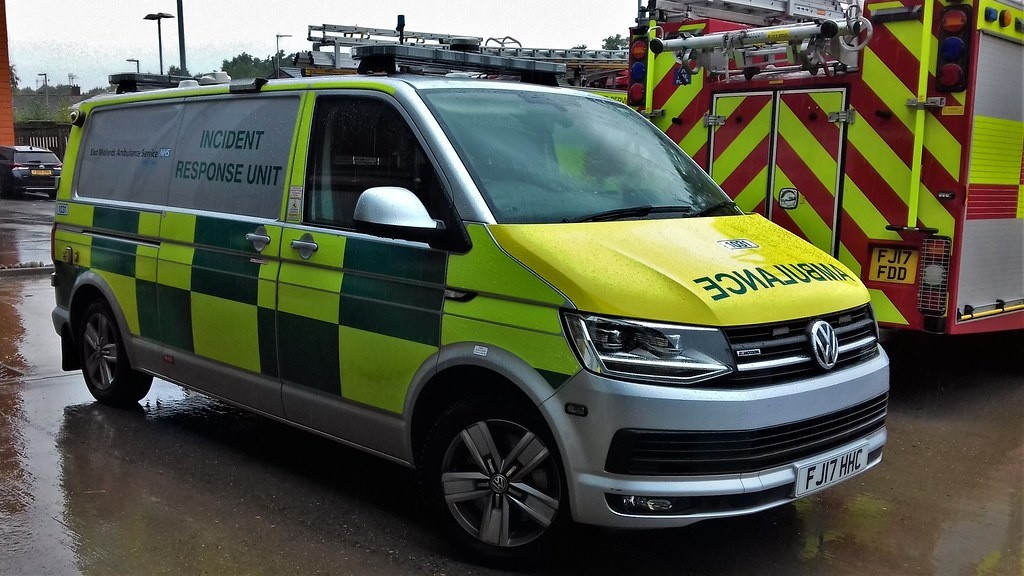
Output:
<box><xmin>309</xmin><ymin>148</ymin><xmax>401</xmax><ymax>223</ymax></box>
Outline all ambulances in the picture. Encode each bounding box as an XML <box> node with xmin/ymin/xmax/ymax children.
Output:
<box><xmin>50</xmin><ymin>44</ymin><xmax>889</xmax><ymax>567</ymax></box>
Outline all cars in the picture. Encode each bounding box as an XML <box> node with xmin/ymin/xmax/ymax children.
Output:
<box><xmin>0</xmin><ymin>145</ymin><xmax>63</xmax><ymax>199</ymax></box>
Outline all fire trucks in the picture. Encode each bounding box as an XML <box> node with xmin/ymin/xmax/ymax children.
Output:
<box><xmin>294</xmin><ymin>0</ymin><xmax>1023</xmax><ymax>337</ymax></box>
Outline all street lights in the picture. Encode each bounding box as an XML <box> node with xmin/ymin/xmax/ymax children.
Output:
<box><xmin>143</xmin><ymin>12</ymin><xmax>175</xmax><ymax>75</ymax></box>
<box><xmin>126</xmin><ymin>59</ymin><xmax>139</xmax><ymax>73</ymax></box>
<box><xmin>276</xmin><ymin>34</ymin><xmax>292</xmax><ymax>80</ymax></box>
<box><xmin>38</xmin><ymin>74</ymin><xmax>47</xmax><ymax>87</ymax></box>
